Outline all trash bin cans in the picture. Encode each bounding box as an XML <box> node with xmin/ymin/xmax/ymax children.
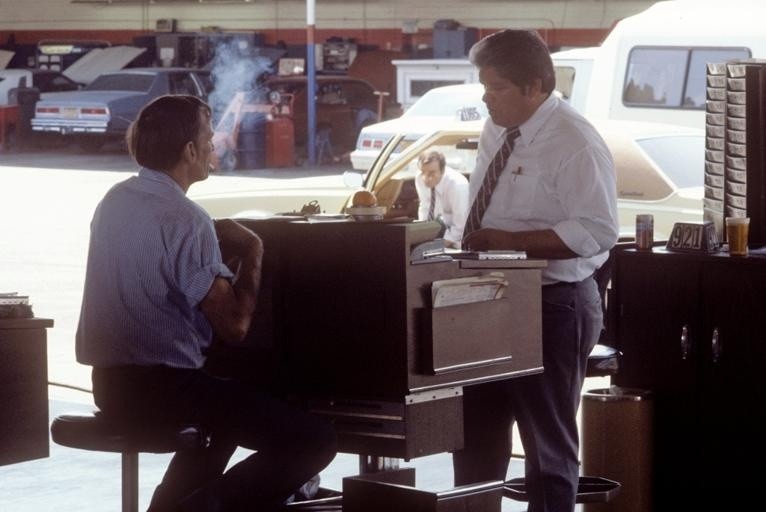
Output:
<box><xmin>582</xmin><ymin>386</ymin><xmax>654</xmax><ymax>512</ymax></box>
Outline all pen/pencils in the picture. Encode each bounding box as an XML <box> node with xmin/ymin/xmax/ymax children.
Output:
<box><xmin>512</xmin><ymin>166</ymin><xmax>521</xmax><ymax>182</ymax></box>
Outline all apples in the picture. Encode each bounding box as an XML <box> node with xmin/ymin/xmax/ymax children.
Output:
<box><xmin>352</xmin><ymin>191</ymin><xmax>375</xmax><ymax>207</ymax></box>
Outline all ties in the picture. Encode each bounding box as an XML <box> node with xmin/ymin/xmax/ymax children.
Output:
<box><xmin>463</xmin><ymin>131</ymin><xmax>520</xmax><ymax>236</ymax></box>
<box><xmin>426</xmin><ymin>188</ymin><xmax>436</xmax><ymax>222</ymax></box>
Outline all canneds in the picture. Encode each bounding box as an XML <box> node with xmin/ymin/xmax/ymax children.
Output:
<box><xmin>636</xmin><ymin>214</ymin><xmax>654</xmax><ymax>251</ymax></box>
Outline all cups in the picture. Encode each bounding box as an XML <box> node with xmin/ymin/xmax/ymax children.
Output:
<box><xmin>725</xmin><ymin>216</ymin><xmax>751</xmax><ymax>256</ymax></box>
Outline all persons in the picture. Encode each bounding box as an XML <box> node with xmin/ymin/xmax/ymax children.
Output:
<box><xmin>412</xmin><ymin>148</ymin><xmax>470</xmax><ymax>248</ymax></box>
<box><xmin>455</xmin><ymin>28</ymin><xmax>619</xmax><ymax>511</ymax></box>
<box><xmin>74</xmin><ymin>93</ymin><xmax>336</xmax><ymax>511</ymax></box>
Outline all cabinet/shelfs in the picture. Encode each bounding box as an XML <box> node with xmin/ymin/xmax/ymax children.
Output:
<box><xmin>615</xmin><ymin>241</ymin><xmax>766</xmax><ymax>511</ymax></box>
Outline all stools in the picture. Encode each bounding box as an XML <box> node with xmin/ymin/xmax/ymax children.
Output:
<box><xmin>503</xmin><ymin>338</ymin><xmax>621</xmax><ymax>504</ymax></box>
<box><xmin>51</xmin><ymin>413</ymin><xmax>199</xmax><ymax>511</ymax></box>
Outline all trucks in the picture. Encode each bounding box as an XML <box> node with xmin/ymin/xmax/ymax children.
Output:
<box><xmin>535</xmin><ymin>1</ymin><xmax>766</xmax><ymax>243</ymax></box>
